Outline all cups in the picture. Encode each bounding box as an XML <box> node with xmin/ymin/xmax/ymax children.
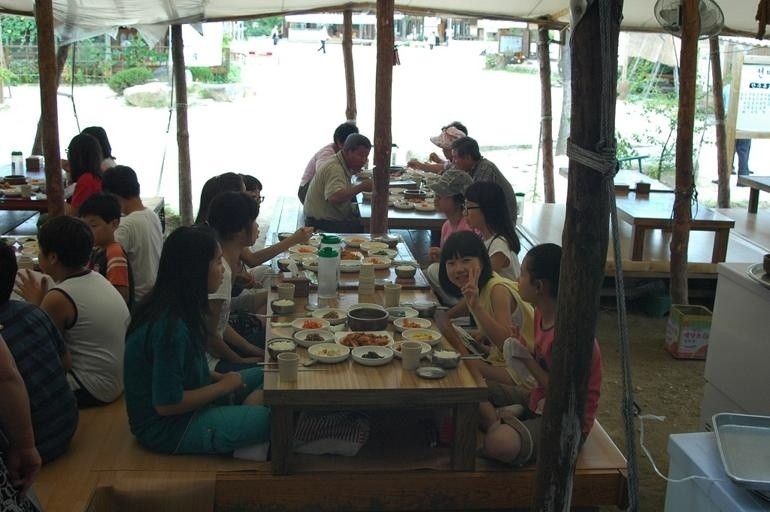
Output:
<box><xmin>401</xmin><ymin>343</ymin><xmax>422</xmax><ymax>371</ymax></box>
<box><xmin>383</xmin><ymin>283</ymin><xmax>402</xmax><ymax>306</ymax></box>
<box><xmin>277</xmin><ymin>282</ymin><xmax>296</xmax><ymax>299</ymax></box>
<box><xmin>279</xmin><ymin>353</ymin><xmax>299</xmax><ymax>383</ymax></box>
<box><xmin>21</xmin><ymin>185</ymin><xmax>32</xmax><ymax>199</ymax></box>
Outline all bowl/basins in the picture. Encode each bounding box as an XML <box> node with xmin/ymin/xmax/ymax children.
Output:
<box><xmin>362</xmin><ymin>192</ymin><xmax>373</xmax><ymax>200</ymax></box>
<box><xmin>277</xmin><ymin>258</ymin><xmax>298</xmax><ymax>272</ymax></box>
<box><xmin>271</xmin><ymin>298</ymin><xmax>295</xmax><ymax>314</ymax></box>
<box><xmin>404</xmin><ymin>189</ymin><xmax>426</xmax><ymax>203</ymax></box>
<box><xmin>432</xmin><ymin>348</ymin><xmax>460</xmax><ymax>369</ymax></box>
<box><xmin>266</xmin><ymin>337</ymin><xmax>298</xmax><ymax>359</ymax></box>
<box><xmin>278</xmin><ymin>232</ymin><xmax>294</xmax><ymax>241</ymax></box>
<box><xmin>32</xmin><ymin>185</ymin><xmax>40</xmax><ymax>191</ymax></box>
<box><xmin>23</xmin><ymin>241</ymin><xmax>39</xmax><ymax>249</ymax></box>
<box><xmin>347</xmin><ymin>309</ymin><xmax>388</xmax><ymax>332</ymax></box>
<box><xmin>395</xmin><ymin>264</ymin><xmax>416</xmax><ymax>279</ymax></box>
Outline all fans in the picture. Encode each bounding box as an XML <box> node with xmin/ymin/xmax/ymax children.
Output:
<box><xmin>653</xmin><ymin>3</ymin><xmax>724</xmax><ymax>39</ymax></box>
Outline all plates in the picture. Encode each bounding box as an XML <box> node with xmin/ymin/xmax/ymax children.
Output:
<box><xmin>386</xmin><ymin>308</ymin><xmax>417</xmax><ymax>320</ymax></box>
<box><xmin>2</xmin><ymin>189</ymin><xmax>21</xmax><ymax>196</ymax></box>
<box><xmin>417</xmin><ymin>366</ymin><xmax>446</xmax><ymax>378</ymax></box>
<box><xmin>352</xmin><ymin>345</ymin><xmax>394</xmax><ymax>366</ymax></box>
<box><xmin>303</xmin><ymin>259</ymin><xmax>320</xmax><ymax>272</ymax></box>
<box><xmin>368</xmin><ymin>248</ymin><xmax>397</xmax><ymax>258</ymax></box>
<box><xmin>402</xmin><ymin>329</ymin><xmax>441</xmax><ymax>346</ymax></box>
<box><xmin>359</xmin><ymin>242</ymin><xmax>389</xmax><ymax>252</ymax></box>
<box><xmin>389</xmin><ymin>188</ymin><xmax>407</xmax><ymax>194</ymax></box>
<box><xmin>312</xmin><ymin>308</ymin><xmax>348</xmax><ymax>323</ymax></box>
<box><xmin>394</xmin><ymin>200</ymin><xmax>413</xmax><ymax>209</ymax></box>
<box><xmin>342</xmin><ymin>251</ymin><xmax>364</xmax><ymax>262</ymax></box>
<box><xmin>388</xmin><ymin>195</ymin><xmax>404</xmax><ymax>206</ymax></box>
<box><xmin>307</xmin><ymin>343</ymin><xmax>350</xmax><ymax>363</ymax></box>
<box><xmin>335</xmin><ymin>330</ymin><xmax>394</xmax><ymax>349</ymax></box>
<box><xmin>288</xmin><ymin>253</ymin><xmax>318</xmax><ymax>264</ymax></box>
<box><xmin>414</xmin><ymin>201</ymin><xmax>435</xmax><ymax>211</ymax></box>
<box><xmin>294</xmin><ymin>330</ymin><xmax>335</xmax><ymax>348</ymax></box>
<box><xmin>340</xmin><ymin>261</ymin><xmax>361</xmax><ymax>273</ymax></box>
<box><xmin>344</xmin><ymin>236</ymin><xmax>369</xmax><ymax>248</ymax></box>
<box><xmin>365</xmin><ymin>258</ymin><xmax>391</xmax><ymax>269</ymax></box>
<box><xmin>390</xmin><ymin>341</ymin><xmax>431</xmax><ymax>360</ymax></box>
<box><xmin>21</xmin><ymin>248</ymin><xmax>41</xmax><ymax>256</ymax></box>
<box><xmin>425</xmin><ymin>197</ymin><xmax>434</xmax><ymax>203</ymax></box>
<box><xmin>392</xmin><ymin>317</ymin><xmax>432</xmax><ymax>333</ymax></box>
<box><xmin>347</xmin><ymin>304</ymin><xmax>384</xmax><ymax>310</ymax></box>
<box><xmin>291</xmin><ymin>318</ymin><xmax>331</xmax><ymax>333</ymax></box>
<box><xmin>288</xmin><ymin>245</ymin><xmax>318</xmax><ymax>253</ymax></box>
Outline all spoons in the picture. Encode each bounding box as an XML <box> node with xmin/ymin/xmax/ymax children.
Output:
<box><xmin>300</xmin><ymin>357</ymin><xmax>318</xmax><ymax>367</ymax></box>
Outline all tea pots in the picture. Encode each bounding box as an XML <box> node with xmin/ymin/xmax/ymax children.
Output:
<box><xmin>11</xmin><ymin>151</ymin><xmax>24</xmax><ymax>176</ymax></box>
<box><xmin>319</xmin><ymin>233</ymin><xmax>341</xmax><ymax>289</ymax></box>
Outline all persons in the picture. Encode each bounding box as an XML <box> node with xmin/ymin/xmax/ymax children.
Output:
<box><xmin>102</xmin><ymin>165</ymin><xmax>164</xmax><ymax>315</ymax></box>
<box><xmin>711</xmin><ymin>83</ymin><xmax>754</xmax><ymax>187</ymax></box>
<box><xmin>0</xmin><ymin>237</ymin><xmax>80</xmax><ymax>466</ymax></box>
<box><xmin>271</xmin><ymin>25</ymin><xmax>279</xmax><ymax>45</ymax></box>
<box><xmin>232</xmin><ymin>169</ymin><xmax>314</xmax><ymax>301</ymax></box>
<box><xmin>475</xmin><ymin>243</ymin><xmax>601</xmax><ymax>465</ymax></box>
<box><xmin>438</xmin><ymin>230</ymin><xmax>537</xmax><ymax>363</ymax></box>
<box><xmin>197</xmin><ymin>173</ymin><xmax>267</xmax><ymax>339</ymax></box>
<box><xmin>428</xmin><ymin>121</ymin><xmax>467</xmax><ymax>164</ymax></box>
<box><xmin>451</xmin><ymin>138</ymin><xmax>518</xmax><ymax>229</ymax></box>
<box><xmin>422</xmin><ymin>171</ymin><xmax>478</xmax><ymax>309</ymax></box>
<box><xmin>77</xmin><ymin>193</ymin><xmax>129</xmax><ymax>304</ymax></box>
<box><xmin>428</xmin><ymin>31</ymin><xmax>435</xmax><ymax>50</ymax></box>
<box><xmin>406</xmin><ymin>127</ymin><xmax>466</xmax><ymax>174</ymax></box>
<box><xmin>0</xmin><ymin>331</ymin><xmax>42</xmax><ymax>512</ymax></box>
<box><xmin>317</xmin><ymin>26</ymin><xmax>331</xmax><ymax>53</ymax></box>
<box><xmin>64</xmin><ymin>134</ymin><xmax>103</xmax><ymax>217</ymax></box>
<box><xmin>303</xmin><ymin>133</ymin><xmax>373</xmax><ymax>232</ymax></box>
<box><xmin>193</xmin><ymin>192</ymin><xmax>266</xmax><ymax>376</ymax></box>
<box><xmin>123</xmin><ymin>226</ymin><xmax>274</xmax><ymax>460</ymax></box>
<box><xmin>62</xmin><ymin>126</ymin><xmax>117</xmax><ymax>176</ymax></box>
<box><xmin>13</xmin><ymin>215</ymin><xmax>132</xmax><ymax>410</ymax></box>
<box><xmin>297</xmin><ymin>123</ymin><xmax>364</xmax><ymax>216</ymax></box>
<box><xmin>441</xmin><ymin>181</ymin><xmax>522</xmax><ymax>332</ymax></box>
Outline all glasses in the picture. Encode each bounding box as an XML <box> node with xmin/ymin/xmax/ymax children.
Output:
<box><xmin>250</xmin><ymin>193</ymin><xmax>264</xmax><ymax>204</ymax></box>
<box><xmin>460</xmin><ymin>202</ymin><xmax>483</xmax><ymax>213</ymax></box>
<box><xmin>63</xmin><ymin>147</ymin><xmax>70</xmax><ymax>153</ymax></box>
<box><xmin>432</xmin><ymin>190</ymin><xmax>456</xmax><ymax>201</ymax></box>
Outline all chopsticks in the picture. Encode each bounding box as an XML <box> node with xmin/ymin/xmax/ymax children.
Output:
<box><xmin>256</xmin><ymin>368</ymin><xmax>329</xmax><ymax>372</ymax></box>
<box><xmin>460</xmin><ymin>353</ymin><xmax>485</xmax><ymax>361</ymax></box>
<box><xmin>251</xmin><ymin>312</ymin><xmax>307</xmax><ymax>318</ymax></box>
<box><xmin>255</xmin><ymin>362</ymin><xmax>305</xmax><ymax>365</ymax></box>
<box><xmin>263</xmin><ymin>272</ymin><xmax>281</xmax><ymax>278</ymax></box>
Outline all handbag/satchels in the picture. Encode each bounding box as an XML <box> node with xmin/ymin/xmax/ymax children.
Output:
<box><xmin>290</xmin><ymin>409</ymin><xmax>372</xmax><ymax>458</ymax></box>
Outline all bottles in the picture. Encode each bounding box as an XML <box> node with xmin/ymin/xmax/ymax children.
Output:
<box><xmin>320</xmin><ymin>233</ymin><xmax>341</xmax><ymax>282</ymax></box>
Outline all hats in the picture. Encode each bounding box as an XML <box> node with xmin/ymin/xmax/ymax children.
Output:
<box><xmin>428</xmin><ymin>126</ymin><xmax>468</xmax><ymax>151</ymax></box>
<box><xmin>430</xmin><ymin>169</ymin><xmax>475</xmax><ymax>198</ymax></box>
